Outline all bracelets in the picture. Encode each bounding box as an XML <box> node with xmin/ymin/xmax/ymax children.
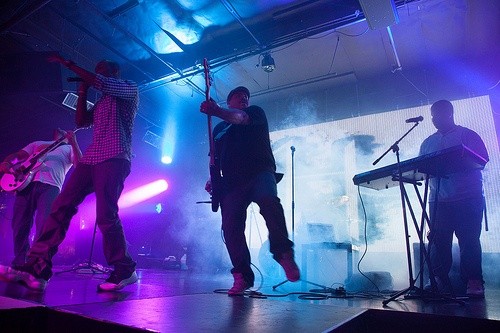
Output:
<box><xmin>67</xmin><ymin>61</ymin><xmax>73</xmax><ymax>67</ymax></box>
<box><xmin>78</xmin><ymin>91</ymin><xmax>87</xmax><ymax>93</ymax></box>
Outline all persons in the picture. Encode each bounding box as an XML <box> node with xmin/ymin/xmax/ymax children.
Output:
<box><xmin>0</xmin><ymin>52</ymin><xmax>139</xmax><ymax>291</ymax></box>
<box><xmin>0</xmin><ymin>127</ymin><xmax>83</xmax><ymax>275</ymax></box>
<box><xmin>200</xmin><ymin>86</ymin><xmax>300</xmax><ymax>295</ymax></box>
<box><xmin>420</xmin><ymin>100</ymin><xmax>490</xmax><ymax>297</ymax></box>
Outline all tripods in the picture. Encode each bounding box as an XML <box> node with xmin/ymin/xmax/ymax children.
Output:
<box><xmin>55</xmin><ymin>221</ymin><xmax>109</xmax><ymax>274</ymax></box>
<box><xmin>372</xmin><ymin>121</ymin><xmax>470</xmax><ymax>307</ymax></box>
<box><xmin>273</xmin><ymin>151</ymin><xmax>331</xmax><ymax>289</ymax></box>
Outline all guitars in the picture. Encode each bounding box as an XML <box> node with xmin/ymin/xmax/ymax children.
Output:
<box><xmin>0</xmin><ymin>124</ymin><xmax>94</xmax><ymax>193</ymax></box>
<box><xmin>203</xmin><ymin>58</ymin><xmax>222</xmax><ymax>213</ymax></box>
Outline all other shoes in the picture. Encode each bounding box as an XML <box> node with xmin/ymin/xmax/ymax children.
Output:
<box><xmin>466</xmin><ymin>280</ymin><xmax>483</xmax><ymax>294</ymax></box>
<box><xmin>425</xmin><ymin>277</ymin><xmax>439</xmax><ymax>289</ymax></box>
<box><xmin>278</xmin><ymin>255</ymin><xmax>299</xmax><ymax>281</ymax></box>
<box><xmin>228</xmin><ymin>273</ymin><xmax>249</xmax><ymax>295</ymax></box>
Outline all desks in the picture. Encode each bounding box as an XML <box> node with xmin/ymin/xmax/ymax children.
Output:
<box><xmin>300</xmin><ymin>241</ymin><xmax>362</xmax><ymax>288</ymax></box>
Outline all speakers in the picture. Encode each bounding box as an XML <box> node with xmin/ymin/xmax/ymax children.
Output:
<box><xmin>0</xmin><ymin>294</ymin><xmax>157</xmax><ymax>333</ymax></box>
<box><xmin>322</xmin><ymin>308</ymin><xmax>500</xmax><ymax>333</ymax></box>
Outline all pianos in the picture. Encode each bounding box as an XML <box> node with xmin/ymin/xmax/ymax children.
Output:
<box><xmin>352</xmin><ymin>143</ymin><xmax>487</xmax><ymax>305</ymax></box>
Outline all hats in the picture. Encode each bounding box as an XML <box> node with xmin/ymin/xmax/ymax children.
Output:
<box><xmin>227</xmin><ymin>86</ymin><xmax>249</xmax><ymax>101</ymax></box>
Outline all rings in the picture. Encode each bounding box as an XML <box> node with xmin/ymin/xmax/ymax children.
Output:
<box><xmin>203</xmin><ymin>109</ymin><xmax>204</xmax><ymax>111</ymax></box>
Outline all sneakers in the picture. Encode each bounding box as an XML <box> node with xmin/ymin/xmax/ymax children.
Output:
<box><xmin>98</xmin><ymin>262</ymin><xmax>139</xmax><ymax>291</ymax></box>
<box><xmin>0</xmin><ymin>264</ymin><xmax>47</xmax><ymax>291</ymax></box>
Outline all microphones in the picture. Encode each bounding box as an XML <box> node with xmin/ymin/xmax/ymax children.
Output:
<box><xmin>406</xmin><ymin>116</ymin><xmax>423</xmax><ymax>123</ymax></box>
<box><xmin>67</xmin><ymin>76</ymin><xmax>83</xmax><ymax>82</ymax></box>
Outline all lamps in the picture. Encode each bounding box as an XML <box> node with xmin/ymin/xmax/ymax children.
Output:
<box><xmin>261</xmin><ymin>51</ymin><xmax>276</xmax><ymax>73</ymax></box>
